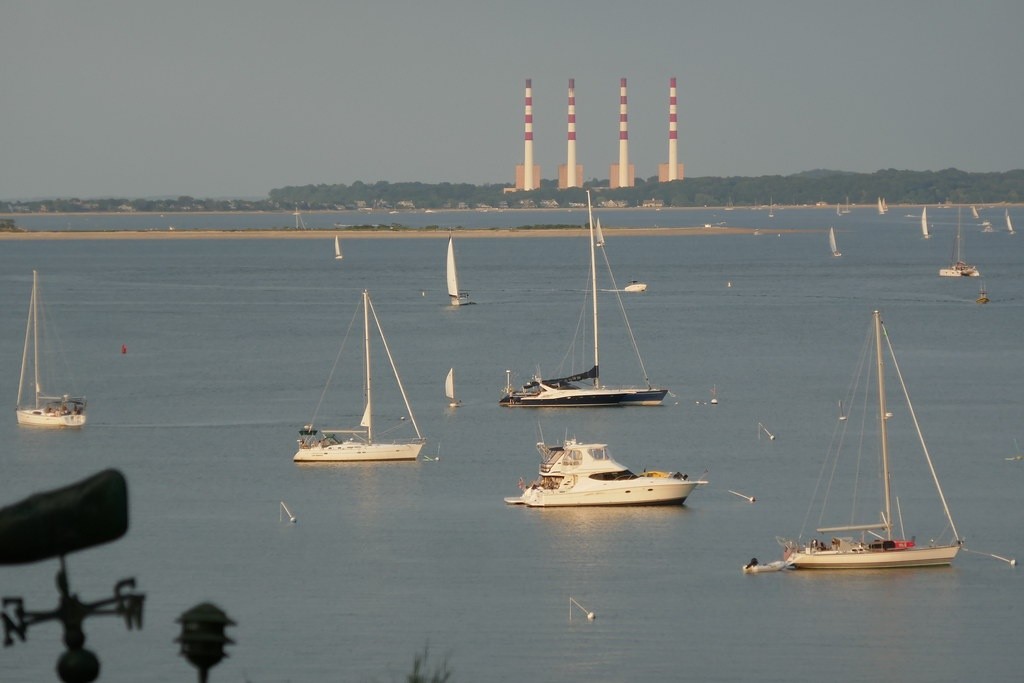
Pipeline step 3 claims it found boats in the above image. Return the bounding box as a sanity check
[504,422,708,508]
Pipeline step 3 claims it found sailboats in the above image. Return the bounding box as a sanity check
[293,289,426,462]
[921,206,934,238]
[445,229,473,305]
[785,309,963,570]
[499,190,668,408]
[334,234,343,260]
[445,368,461,407]
[877,197,888,215]
[828,227,842,257]
[939,210,979,277]
[14,270,86,429]
